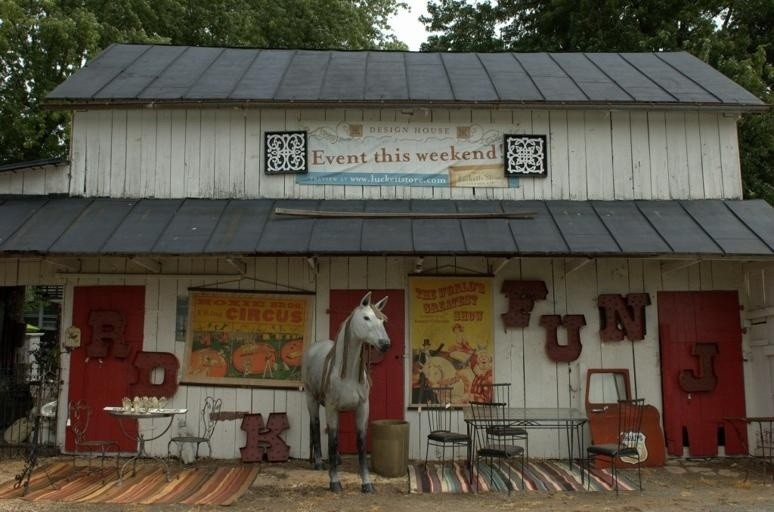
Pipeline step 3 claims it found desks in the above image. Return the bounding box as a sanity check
[729,414,774,483]
[104,406,188,484]
[464,407,588,484]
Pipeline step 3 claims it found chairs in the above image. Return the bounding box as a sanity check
[167,396,222,477]
[421,382,530,494]
[588,398,645,498]
[67,397,120,476]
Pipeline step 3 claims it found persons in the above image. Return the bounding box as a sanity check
[418,323,493,404]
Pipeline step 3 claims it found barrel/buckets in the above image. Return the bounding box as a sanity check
[371,418,410,478]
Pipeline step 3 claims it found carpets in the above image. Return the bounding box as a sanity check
[0,460,259,506]
[404,459,641,494]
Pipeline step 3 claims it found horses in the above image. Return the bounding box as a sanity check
[300,291,391,494]
